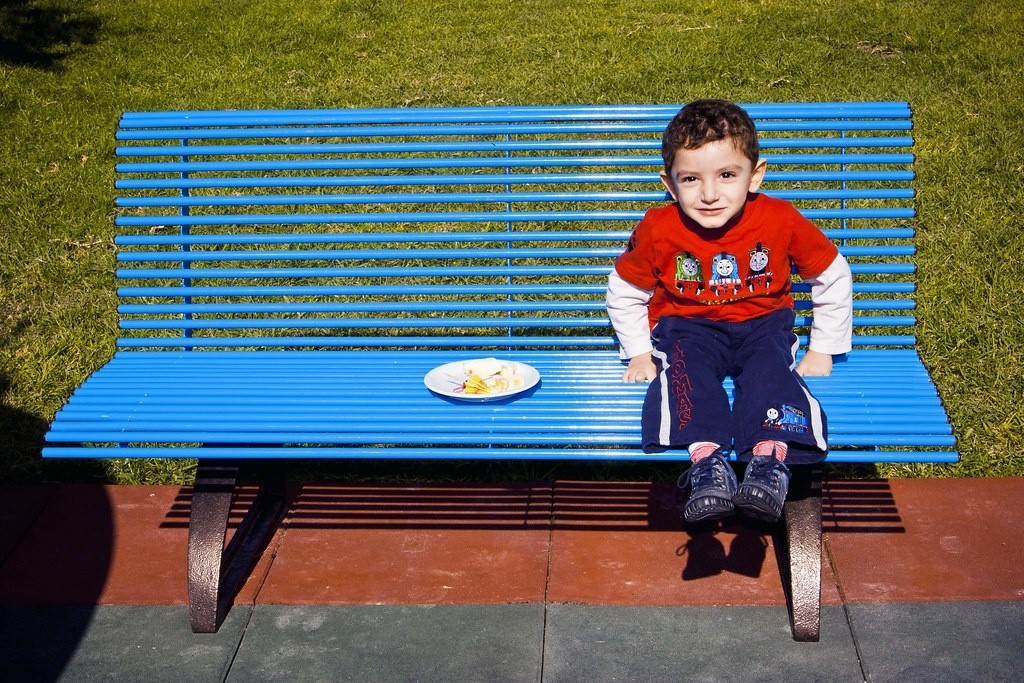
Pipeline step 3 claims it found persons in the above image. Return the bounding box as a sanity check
[606,100,853,523]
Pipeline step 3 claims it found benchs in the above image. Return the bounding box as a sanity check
[42,103,960,644]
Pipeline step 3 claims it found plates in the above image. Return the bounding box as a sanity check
[423,359,540,402]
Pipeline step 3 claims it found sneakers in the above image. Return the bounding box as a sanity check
[731,443,792,523]
[677,445,738,522]
